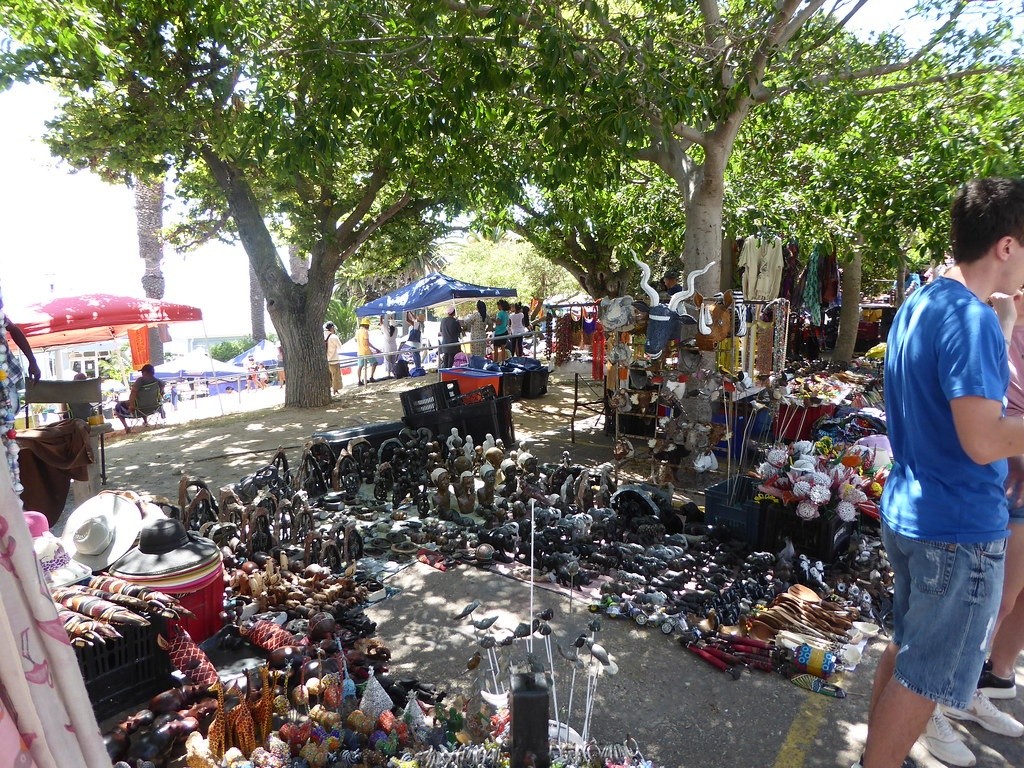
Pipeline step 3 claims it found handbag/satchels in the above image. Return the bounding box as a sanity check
[408,323,420,343]
[410,367,426,377]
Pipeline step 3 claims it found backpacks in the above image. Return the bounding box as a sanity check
[395,360,410,379]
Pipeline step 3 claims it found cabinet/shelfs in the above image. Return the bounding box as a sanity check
[616,298,733,452]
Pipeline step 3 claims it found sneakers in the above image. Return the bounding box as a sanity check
[918,707,977,767]
[942,689,1024,737]
[977,659,1017,698]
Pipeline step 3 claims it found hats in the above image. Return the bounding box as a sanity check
[106,517,225,594]
[31,531,93,589]
[23,511,49,538]
[138,365,155,375]
[62,492,141,571]
[447,308,455,315]
[360,318,370,325]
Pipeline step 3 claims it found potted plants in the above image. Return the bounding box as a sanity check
[87,408,103,425]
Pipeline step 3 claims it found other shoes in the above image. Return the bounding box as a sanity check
[851,751,917,768]
[143,422,147,427]
[358,381,364,385]
[126,427,131,434]
[370,378,378,382]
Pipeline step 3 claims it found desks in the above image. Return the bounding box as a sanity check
[14,422,114,510]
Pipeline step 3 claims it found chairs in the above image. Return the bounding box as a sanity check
[121,381,164,432]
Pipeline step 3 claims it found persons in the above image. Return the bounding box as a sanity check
[245,354,269,391]
[437,307,466,369]
[171,385,178,410]
[324,321,343,396]
[0,287,41,443]
[397,310,426,369]
[226,386,237,394]
[379,314,398,378]
[354,318,377,386]
[275,341,285,388]
[850,173,1024,768]
[430,428,616,517]
[490,299,533,366]
[61,373,96,422]
[663,270,683,296]
[466,300,494,370]
[114,364,161,434]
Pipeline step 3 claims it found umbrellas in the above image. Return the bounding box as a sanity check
[6,285,202,484]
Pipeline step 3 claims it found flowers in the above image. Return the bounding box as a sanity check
[754,436,889,522]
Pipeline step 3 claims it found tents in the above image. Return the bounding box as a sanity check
[542,291,598,331]
[357,271,518,377]
[129,348,249,410]
[225,338,285,367]
